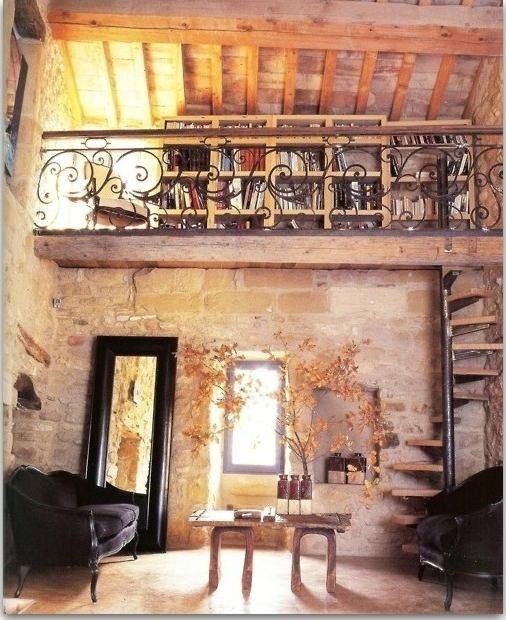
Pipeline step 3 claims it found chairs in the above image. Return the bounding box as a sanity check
[412,463,504,612]
[82,160,151,229]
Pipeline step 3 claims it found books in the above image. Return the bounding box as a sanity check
[217,178,266,210]
[166,122,212,129]
[275,150,324,209]
[162,146,212,171]
[224,122,253,129]
[177,219,202,230]
[221,147,267,171]
[288,219,299,229]
[332,147,382,210]
[162,181,204,210]
[391,133,471,216]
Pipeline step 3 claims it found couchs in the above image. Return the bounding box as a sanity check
[4,466,140,605]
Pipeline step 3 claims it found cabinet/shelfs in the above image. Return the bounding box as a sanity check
[154,112,478,233]
